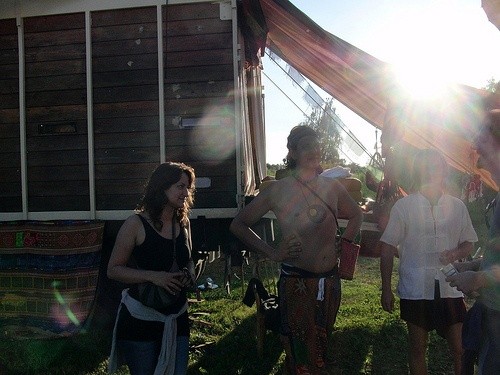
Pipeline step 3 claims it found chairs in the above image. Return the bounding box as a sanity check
[252,257,283,360]
[225,239,265,298]
